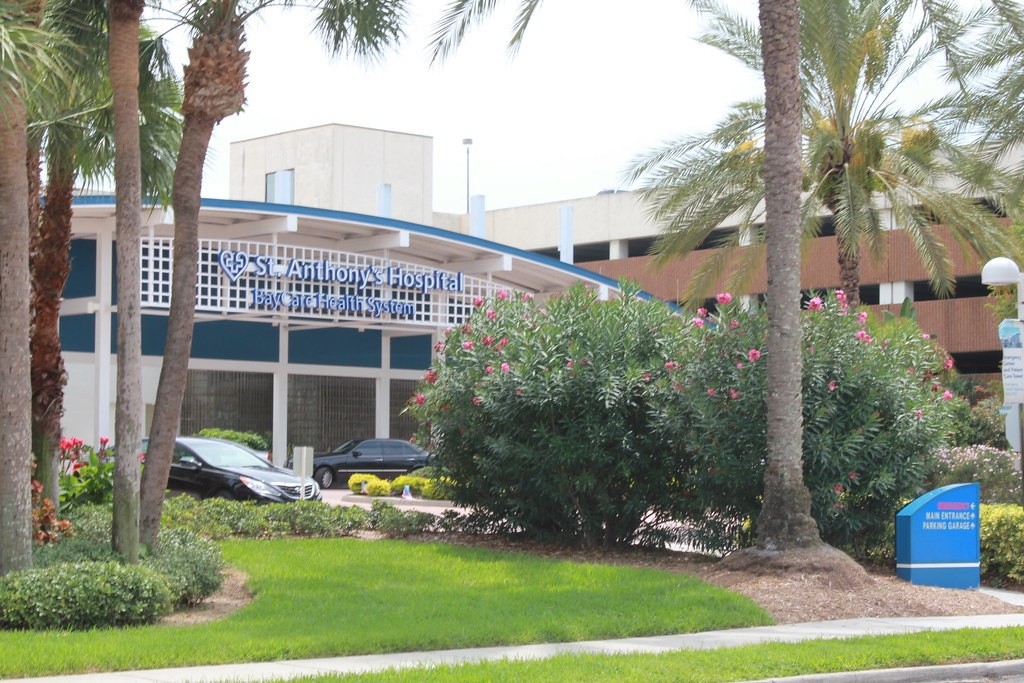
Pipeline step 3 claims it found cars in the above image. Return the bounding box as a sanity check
[95,435,322,507]
[284,439,438,490]
